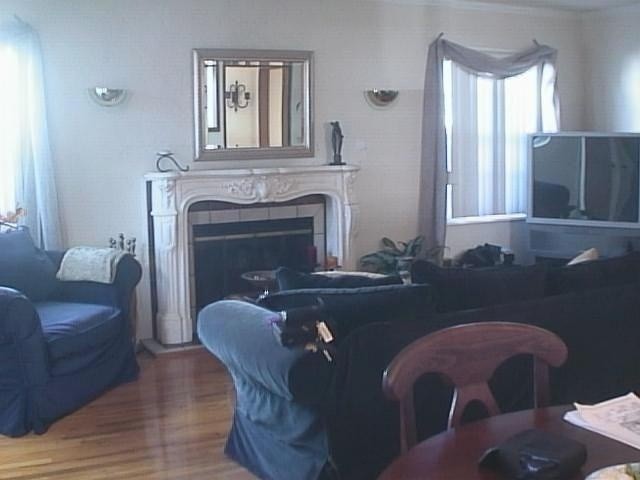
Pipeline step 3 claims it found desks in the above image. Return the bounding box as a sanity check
[374,401,640,480]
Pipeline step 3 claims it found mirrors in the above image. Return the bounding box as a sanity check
[189,43,318,162]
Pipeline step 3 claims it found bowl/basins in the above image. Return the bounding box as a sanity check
[240,270,277,290]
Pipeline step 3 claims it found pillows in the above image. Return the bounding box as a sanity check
[564,245,604,264]
[253,277,436,328]
[269,263,402,294]
[566,250,639,290]
[404,256,604,299]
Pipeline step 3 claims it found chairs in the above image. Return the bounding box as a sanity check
[374,318,572,456]
[0,224,145,445]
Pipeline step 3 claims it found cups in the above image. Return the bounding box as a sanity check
[327,256,337,267]
[307,245,317,265]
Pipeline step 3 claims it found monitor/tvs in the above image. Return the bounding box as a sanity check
[526,131,640,229]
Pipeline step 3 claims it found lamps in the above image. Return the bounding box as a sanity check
[85,86,128,109]
[360,87,401,110]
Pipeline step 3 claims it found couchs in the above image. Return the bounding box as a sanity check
[191,250,640,480]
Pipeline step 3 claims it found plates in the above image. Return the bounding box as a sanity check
[583,460,639,480]
